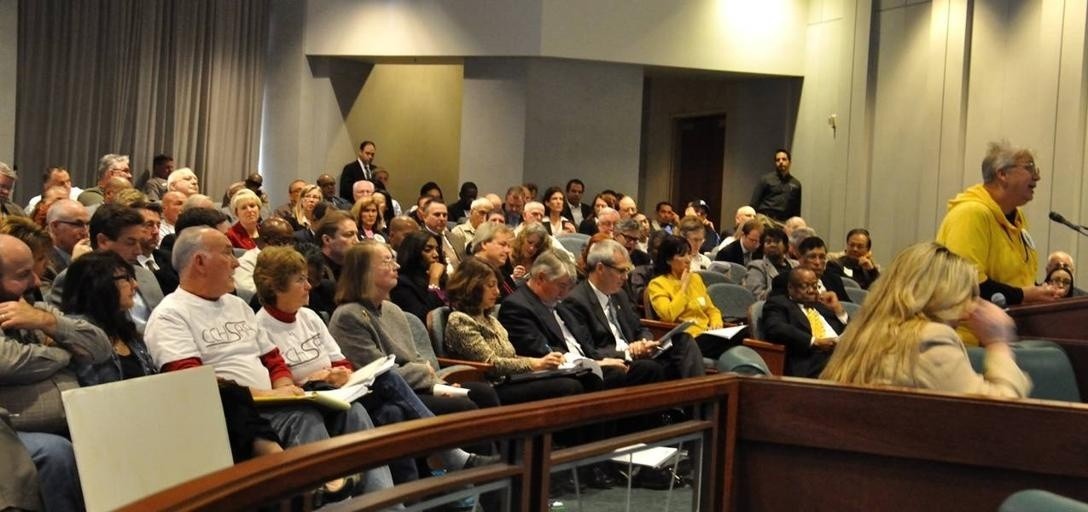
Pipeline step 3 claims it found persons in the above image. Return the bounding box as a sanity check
[815,239,1036,404]
[253,244,500,507]
[928,136,1082,403]
[445,234,853,498]
[1038,266,1075,299]
[328,239,499,457]
[1035,249,1087,298]
[3,139,882,337]
[143,225,406,509]
[3,234,114,510]
[62,250,284,464]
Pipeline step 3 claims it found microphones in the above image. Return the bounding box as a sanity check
[1049,211,1088,237]
[992,292,1010,316]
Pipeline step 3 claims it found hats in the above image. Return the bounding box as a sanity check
[692,199,710,213]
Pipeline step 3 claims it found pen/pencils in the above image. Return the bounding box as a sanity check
[545,344,565,366]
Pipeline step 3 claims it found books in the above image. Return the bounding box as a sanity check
[253,354,397,408]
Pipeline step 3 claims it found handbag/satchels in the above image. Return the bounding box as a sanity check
[608,462,687,490]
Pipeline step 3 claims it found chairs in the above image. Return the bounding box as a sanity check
[425,305,495,376]
[547,228,594,269]
[625,259,868,376]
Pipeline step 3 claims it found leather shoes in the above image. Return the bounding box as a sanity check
[591,476,615,489]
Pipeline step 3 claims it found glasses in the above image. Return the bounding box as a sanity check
[112,271,133,281]
[1051,278,1070,285]
[620,233,640,244]
[748,238,757,244]
[605,264,630,272]
[56,219,85,228]
[1015,163,1039,175]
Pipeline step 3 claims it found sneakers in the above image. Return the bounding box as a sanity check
[463,453,502,469]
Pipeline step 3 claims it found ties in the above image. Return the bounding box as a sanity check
[608,299,630,345]
[804,303,825,338]
[364,166,371,180]
[744,253,750,266]
[439,233,459,269]
